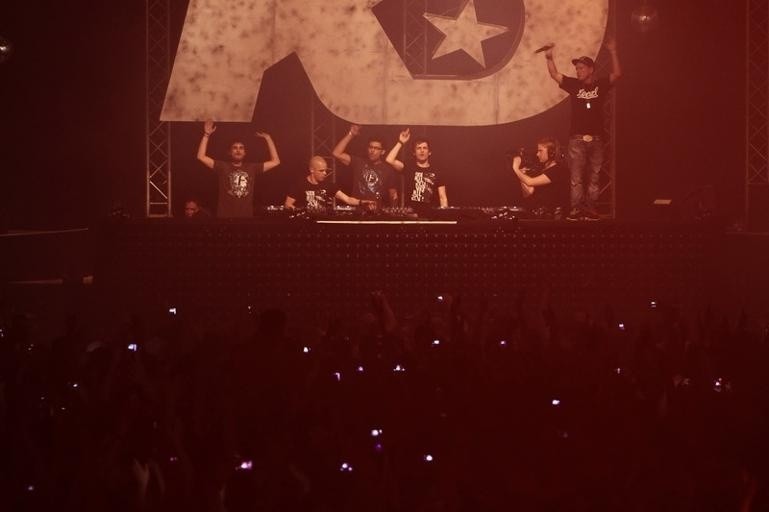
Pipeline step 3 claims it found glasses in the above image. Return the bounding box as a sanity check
[368,146,383,149]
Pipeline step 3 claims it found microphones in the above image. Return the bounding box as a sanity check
[534,42,556,54]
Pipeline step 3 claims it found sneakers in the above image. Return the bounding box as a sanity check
[584,210,601,220]
[567,208,584,221]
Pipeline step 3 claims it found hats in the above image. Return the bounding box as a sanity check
[572,57,595,72]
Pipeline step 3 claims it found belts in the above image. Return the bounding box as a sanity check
[571,135,602,142]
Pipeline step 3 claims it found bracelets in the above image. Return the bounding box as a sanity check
[349,130,354,138]
[359,199,362,206]
[204,131,210,138]
[545,53,553,60]
[397,140,403,146]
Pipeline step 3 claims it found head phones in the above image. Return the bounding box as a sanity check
[548,136,556,162]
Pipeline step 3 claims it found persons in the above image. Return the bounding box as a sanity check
[385,128,448,209]
[182,199,199,217]
[283,156,376,211]
[196,119,280,217]
[332,124,396,209]
[1,286,769,510]
[544,38,622,221]
[512,140,561,208]
